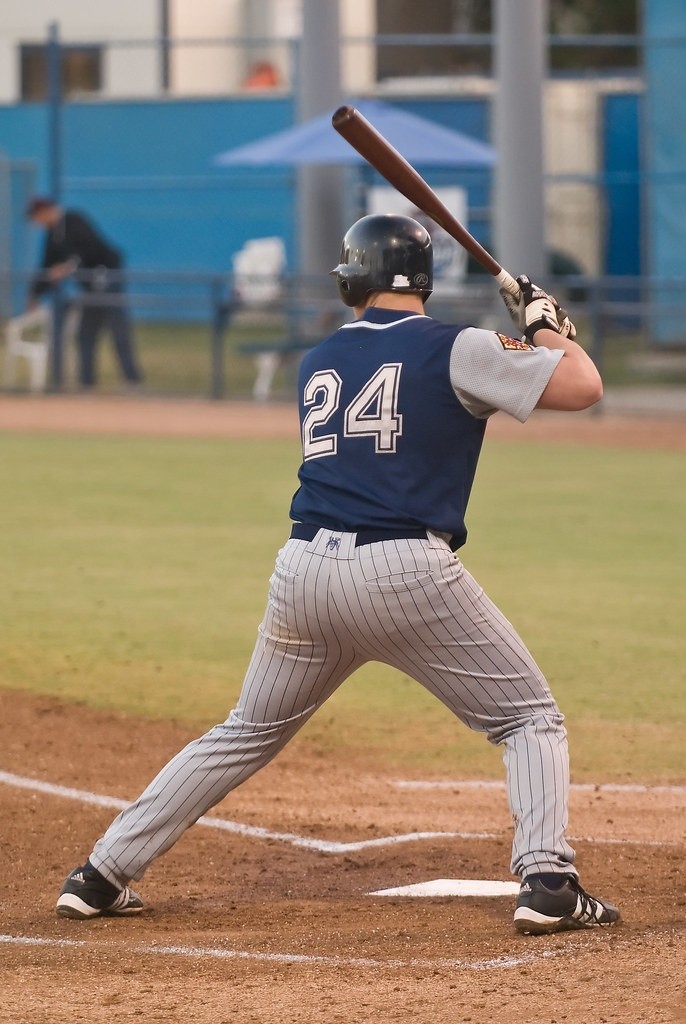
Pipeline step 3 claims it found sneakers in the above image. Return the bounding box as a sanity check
[512,873,620,932]
[55,862,145,918]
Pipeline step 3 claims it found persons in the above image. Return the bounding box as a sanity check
[55,213,625,937]
[24,199,149,390]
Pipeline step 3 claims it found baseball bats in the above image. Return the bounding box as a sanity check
[331,105,524,294]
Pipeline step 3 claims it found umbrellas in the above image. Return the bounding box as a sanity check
[211,95,504,167]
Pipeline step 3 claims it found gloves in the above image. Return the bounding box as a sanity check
[499,276,577,342]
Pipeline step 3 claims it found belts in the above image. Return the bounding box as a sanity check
[289,520,427,550]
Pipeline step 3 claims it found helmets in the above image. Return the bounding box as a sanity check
[329,213,434,306]
